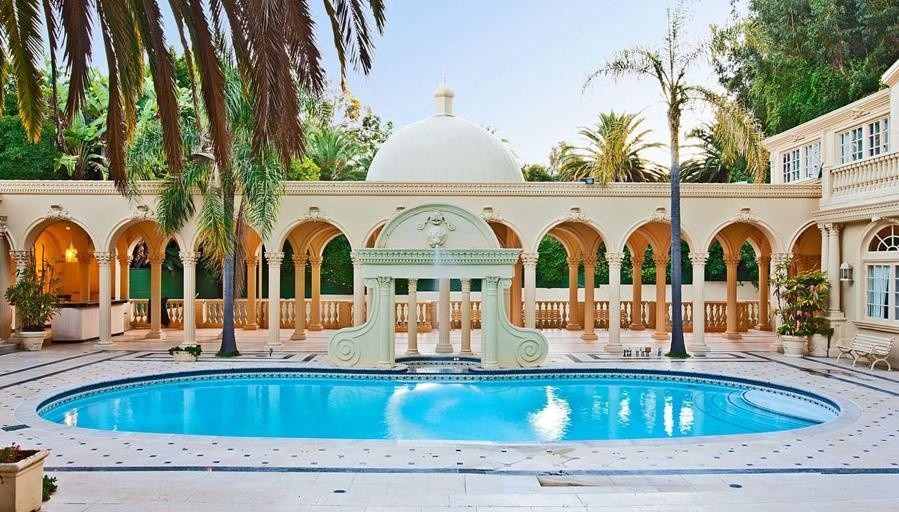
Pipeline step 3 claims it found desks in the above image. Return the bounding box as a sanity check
[50,299,128,343]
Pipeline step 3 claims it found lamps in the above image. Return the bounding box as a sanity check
[838,261,853,283]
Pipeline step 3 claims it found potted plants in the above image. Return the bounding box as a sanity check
[767,251,835,358]
[2,248,66,353]
[0,441,50,511]
[168,345,203,363]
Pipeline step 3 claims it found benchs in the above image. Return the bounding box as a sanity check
[835,331,896,374]
